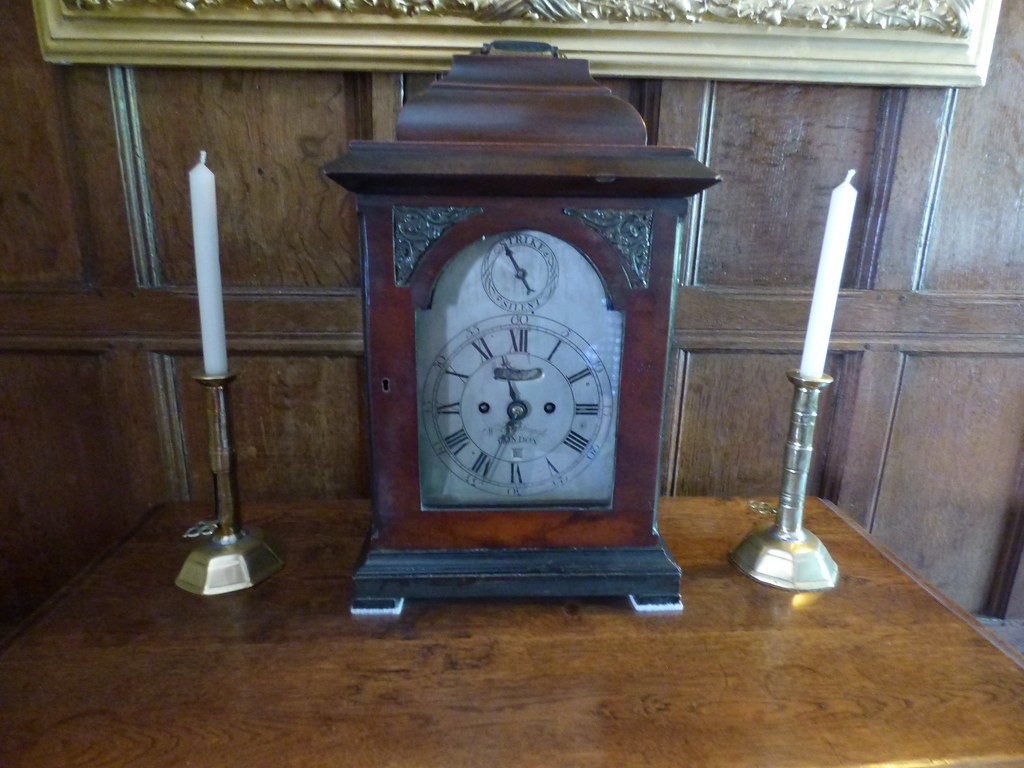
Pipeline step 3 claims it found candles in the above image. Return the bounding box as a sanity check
[188,151,229,377]
[798,169,857,379]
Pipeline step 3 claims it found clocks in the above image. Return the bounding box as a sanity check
[424,231,613,500]
